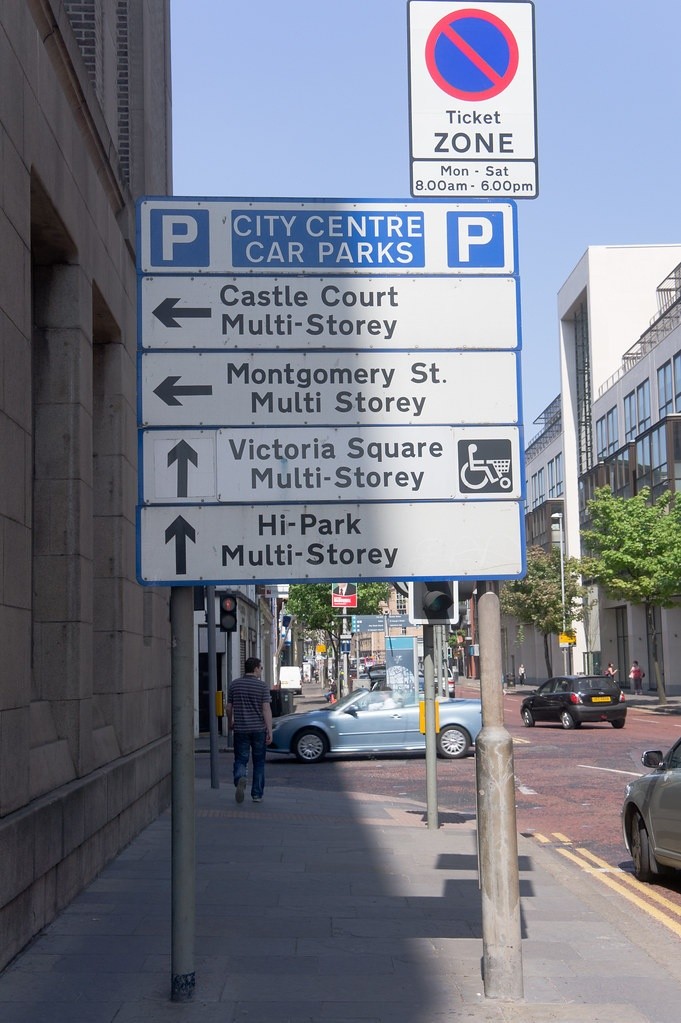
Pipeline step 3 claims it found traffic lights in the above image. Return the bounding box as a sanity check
[219,594,238,632]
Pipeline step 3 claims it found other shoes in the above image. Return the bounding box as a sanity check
[639,690,643,694]
[235,778,247,804]
[253,796,262,802]
[635,690,638,695]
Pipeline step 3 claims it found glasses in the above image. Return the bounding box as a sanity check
[259,667,264,670]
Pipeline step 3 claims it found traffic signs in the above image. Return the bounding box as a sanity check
[133,195,528,587]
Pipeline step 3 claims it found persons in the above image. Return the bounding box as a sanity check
[324,679,337,703]
[605,661,618,679]
[502,673,507,695]
[226,657,273,803]
[630,660,643,695]
[519,663,525,686]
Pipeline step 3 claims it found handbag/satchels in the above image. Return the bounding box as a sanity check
[524,672,526,679]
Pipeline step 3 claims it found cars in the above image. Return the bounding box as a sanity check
[330,657,425,692]
[434,668,455,698]
[520,675,628,730]
[267,689,483,763]
[619,737,681,882]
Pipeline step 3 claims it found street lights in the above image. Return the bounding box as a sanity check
[552,513,567,675]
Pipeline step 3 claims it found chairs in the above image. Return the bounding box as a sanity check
[395,702,404,709]
